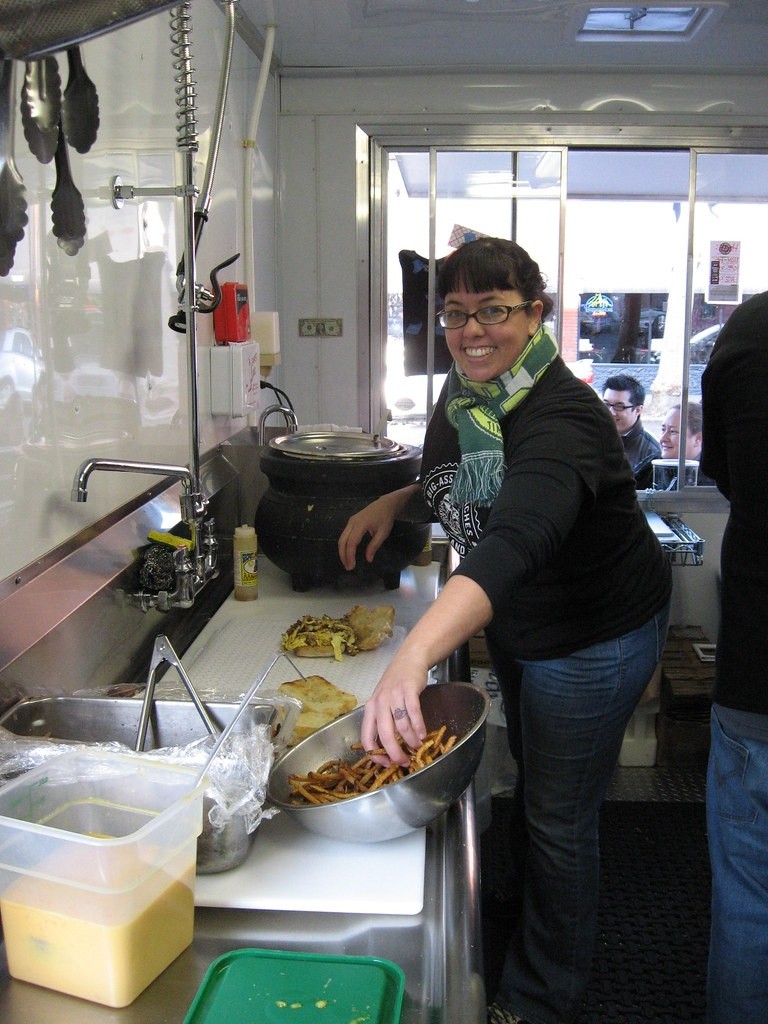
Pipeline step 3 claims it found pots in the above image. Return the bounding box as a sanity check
[255,441,431,592]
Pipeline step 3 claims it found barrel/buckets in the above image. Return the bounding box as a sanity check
[1,750,214,1008]
[651,459,700,492]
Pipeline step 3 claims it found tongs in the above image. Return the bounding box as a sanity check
[50,46,99,257]
[136,634,218,752]
[0,54,62,279]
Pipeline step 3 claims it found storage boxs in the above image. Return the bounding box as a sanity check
[0,752,203,1007]
[0,693,278,881]
[252,432,441,592]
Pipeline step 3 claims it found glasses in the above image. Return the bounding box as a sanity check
[603,403,640,411]
[435,300,534,329]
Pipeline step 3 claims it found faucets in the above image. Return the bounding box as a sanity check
[256,404,301,449]
[70,457,205,518]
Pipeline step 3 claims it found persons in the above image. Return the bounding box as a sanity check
[338,231,682,1020]
[626,400,716,489]
[602,372,666,492]
[696,284,766,1024]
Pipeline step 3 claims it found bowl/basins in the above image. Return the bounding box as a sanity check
[267,681,492,843]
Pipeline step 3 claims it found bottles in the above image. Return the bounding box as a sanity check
[233,524,258,601]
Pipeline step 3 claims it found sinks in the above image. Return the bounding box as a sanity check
[3,691,290,792]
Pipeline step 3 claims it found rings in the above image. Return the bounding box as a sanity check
[392,706,407,722]
